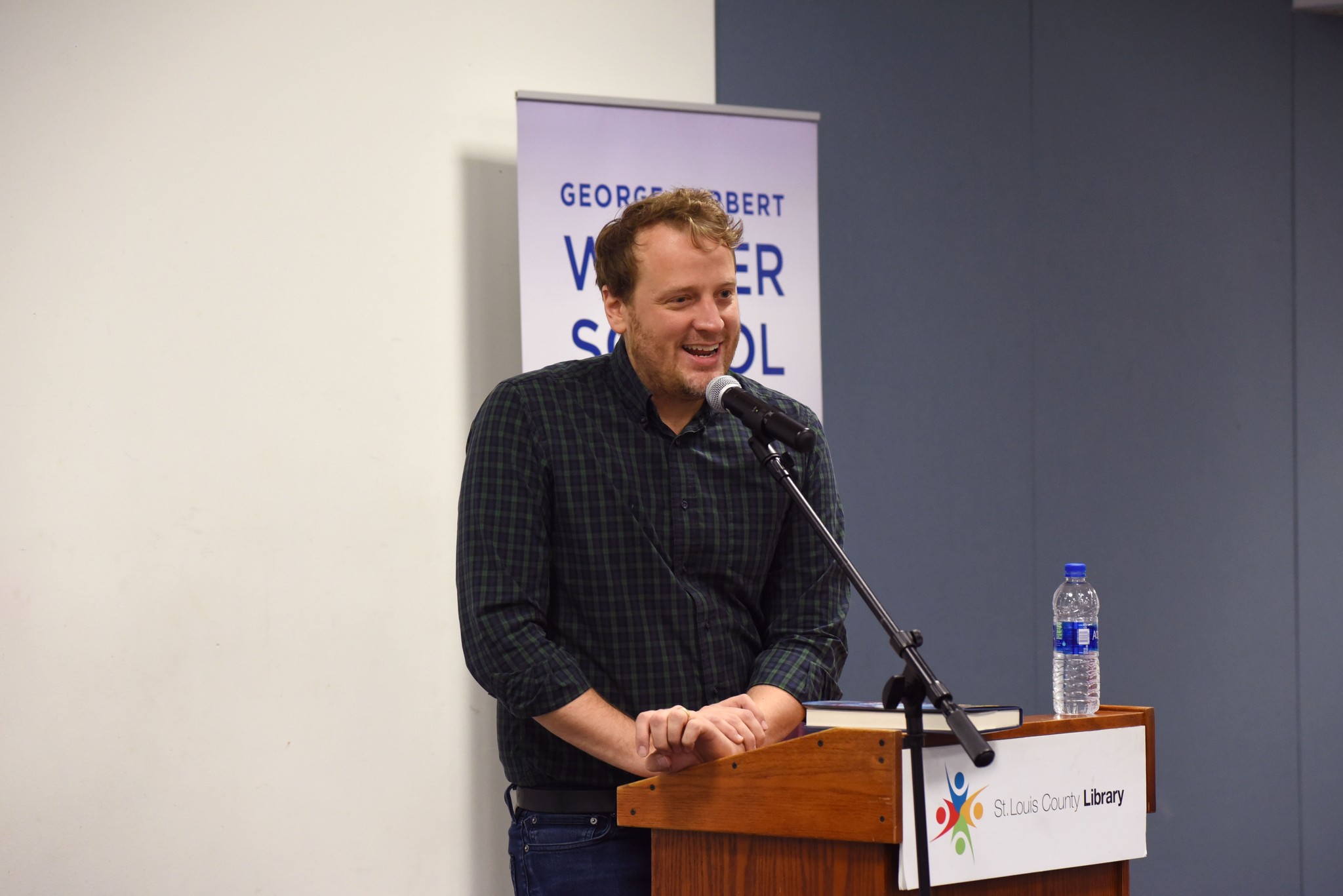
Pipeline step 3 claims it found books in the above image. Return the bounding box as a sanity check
[802,701,1023,737]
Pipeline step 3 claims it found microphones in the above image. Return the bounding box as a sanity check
[706,375,816,453]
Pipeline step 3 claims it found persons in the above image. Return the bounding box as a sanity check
[457,188,848,896]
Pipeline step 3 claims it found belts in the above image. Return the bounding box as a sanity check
[515,785,617,814]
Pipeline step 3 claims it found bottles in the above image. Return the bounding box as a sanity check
[1053,562,1101,716]
